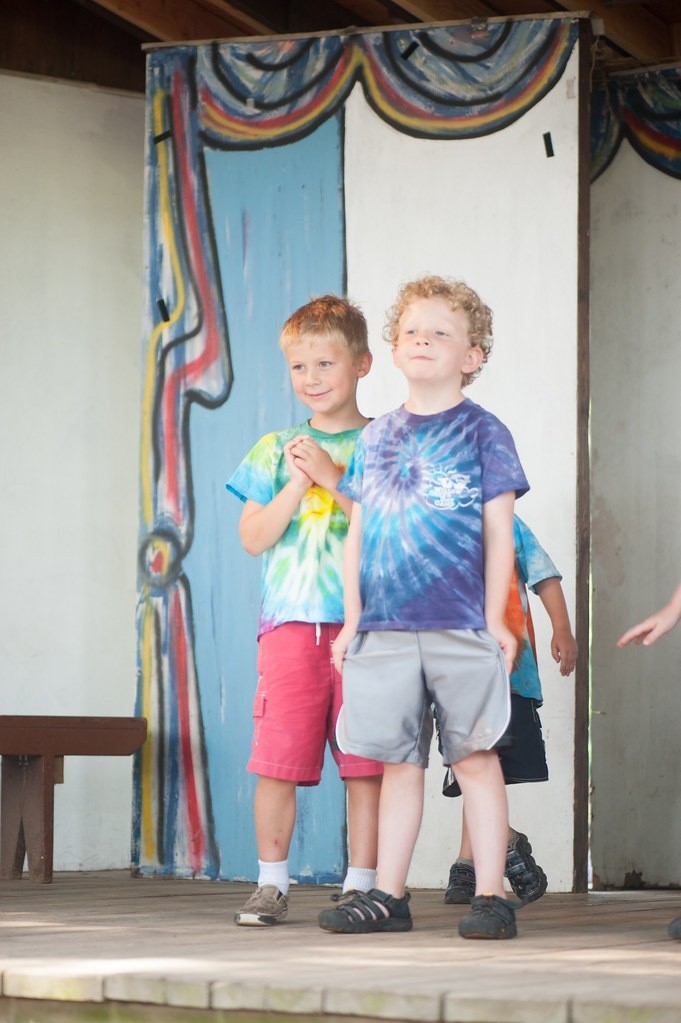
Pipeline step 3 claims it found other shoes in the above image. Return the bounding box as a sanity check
[667,916,681,940]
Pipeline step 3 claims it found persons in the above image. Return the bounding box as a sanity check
[224,273,575,940]
[614,582,681,649]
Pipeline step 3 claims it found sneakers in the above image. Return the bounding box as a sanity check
[337,890,365,907]
[505,831,549,902]
[444,862,476,904]
[234,885,289,926]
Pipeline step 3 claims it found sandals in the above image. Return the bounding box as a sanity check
[458,894,525,939]
[318,888,412,933]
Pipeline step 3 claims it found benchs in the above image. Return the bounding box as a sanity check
[0,714,147,884]
